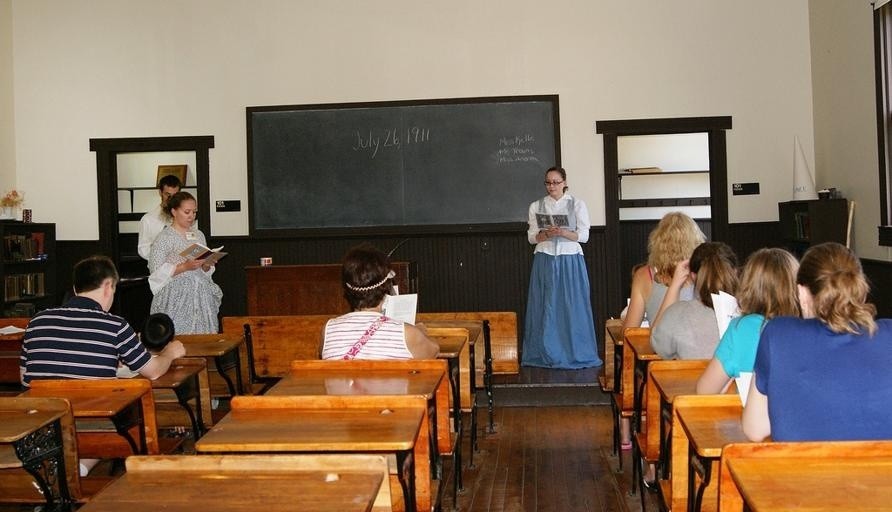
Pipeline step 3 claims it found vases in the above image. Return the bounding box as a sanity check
[0,209,18,219]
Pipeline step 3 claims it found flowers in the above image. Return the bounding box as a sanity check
[2,188,26,208]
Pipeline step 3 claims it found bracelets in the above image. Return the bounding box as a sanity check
[546,231,551,239]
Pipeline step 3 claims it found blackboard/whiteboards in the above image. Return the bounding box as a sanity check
[246,95,561,237]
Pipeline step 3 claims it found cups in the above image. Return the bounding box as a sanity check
[23,208,32,223]
[259,256,273,267]
[818,188,831,198]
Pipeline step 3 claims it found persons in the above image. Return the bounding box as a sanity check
[519,166,604,369]
[621,212,707,338]
[318,248,439,363]
[621,262,648,321]
[741,243,891,443]
[147,191,230,410]
[695,247,800,395]
[19,255,186,477]
[138,175,181,260]
[116,312,175,378]
[649,241,740,360]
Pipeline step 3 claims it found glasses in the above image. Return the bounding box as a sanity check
[544,180,564,186]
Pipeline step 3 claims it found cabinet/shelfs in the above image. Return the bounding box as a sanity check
[1,220,58,315]
[778,198,849,264]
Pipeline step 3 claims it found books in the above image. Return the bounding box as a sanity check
[630,167,664,173]
[710,291,738,340]
[2,233,49,303]
[179,244,228,266]
[384,294,419,324]
[531,213,570,229]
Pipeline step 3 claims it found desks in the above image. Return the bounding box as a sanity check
[80,311,495,509]
[599,310,892,511]
[2,310,247,512]
